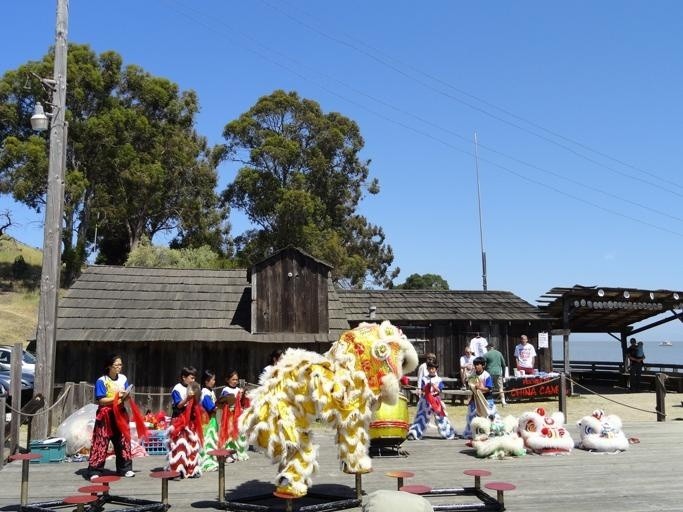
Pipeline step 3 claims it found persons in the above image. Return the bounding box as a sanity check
[514,335,537,375]
[417,353,437,399]
[627,342,647,371]
[218,371,249,462]
[198,369,220,472]
[459,343,476,391]
[482,343,508,407]
[407,357,459,440]
[461,356,499,439]
[164,366,205,481]
[87,355,136,482]
[627,338,641,393]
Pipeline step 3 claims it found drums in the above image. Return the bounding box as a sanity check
[368,394,408,443]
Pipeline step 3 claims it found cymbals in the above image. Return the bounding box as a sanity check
[429,378,432,391]
[215,393,236,409]
[121,384,136,401]
[187,382,201,406]
[241,388,249,407]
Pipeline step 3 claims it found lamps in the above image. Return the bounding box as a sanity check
[594,288,679,302]
[29,99,60,131]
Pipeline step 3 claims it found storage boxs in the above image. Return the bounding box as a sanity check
[30,441,66,463]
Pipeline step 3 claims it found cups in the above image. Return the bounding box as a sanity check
[512,367,559,377]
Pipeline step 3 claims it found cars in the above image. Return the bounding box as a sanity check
[0,343,36,374]
[0,368,35,397]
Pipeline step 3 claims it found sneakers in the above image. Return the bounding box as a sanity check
[225,456,234,463]
[125,471,135,478]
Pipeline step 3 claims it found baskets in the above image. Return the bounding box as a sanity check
[141,435,168,455]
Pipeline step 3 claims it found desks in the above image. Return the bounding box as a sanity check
[505,371,570,402]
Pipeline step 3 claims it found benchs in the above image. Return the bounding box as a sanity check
[621,371,683,393]
[402,374,473,407]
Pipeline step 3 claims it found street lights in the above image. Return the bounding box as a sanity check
[27,100,67,444]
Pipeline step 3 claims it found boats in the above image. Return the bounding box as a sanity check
[657,339,673,347]
